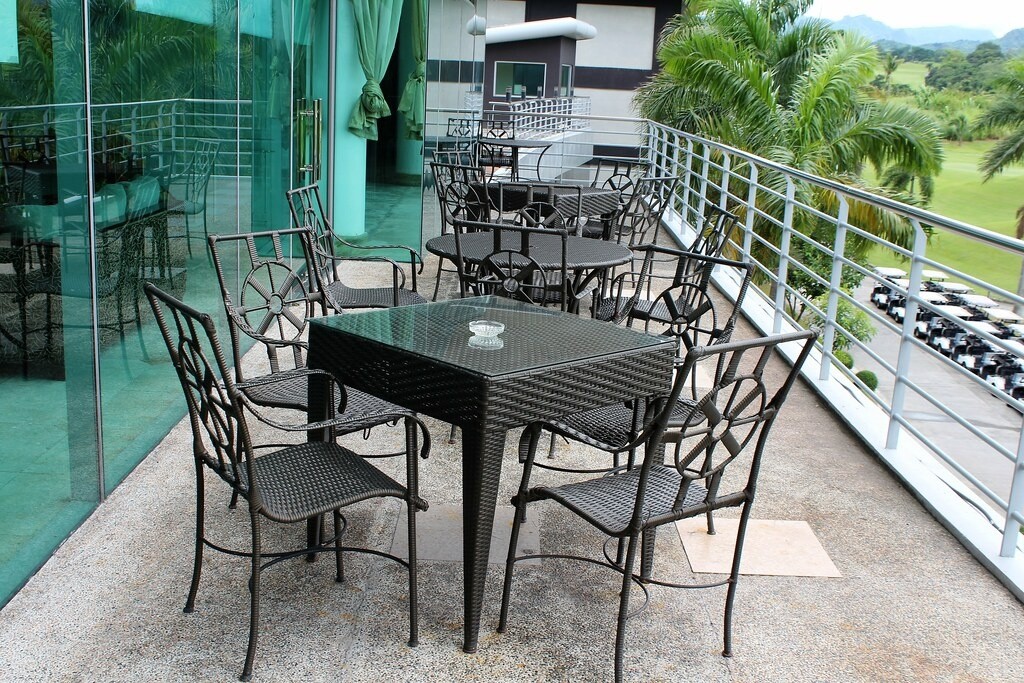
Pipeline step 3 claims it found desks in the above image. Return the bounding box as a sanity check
[479,138,553,186]
[300,295,680,653]
[424,135,478,193]
[463,177,623,311]
[426,231,635,320]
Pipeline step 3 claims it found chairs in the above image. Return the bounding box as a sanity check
[2,128,220,386]
[447,217,573,449]
[578,177,679,253]
[498,327,820,682]
[442,118,482,179]
[286,185,429,317]
[592,204,741,372]
[431,150,499,224]
[495,182,583,305]
[207,227,418,508]
[139,279,430,681]
[543,241,756,477]
[478,118,520,183]
[568,156,650,237]
[430,160,522,280]
